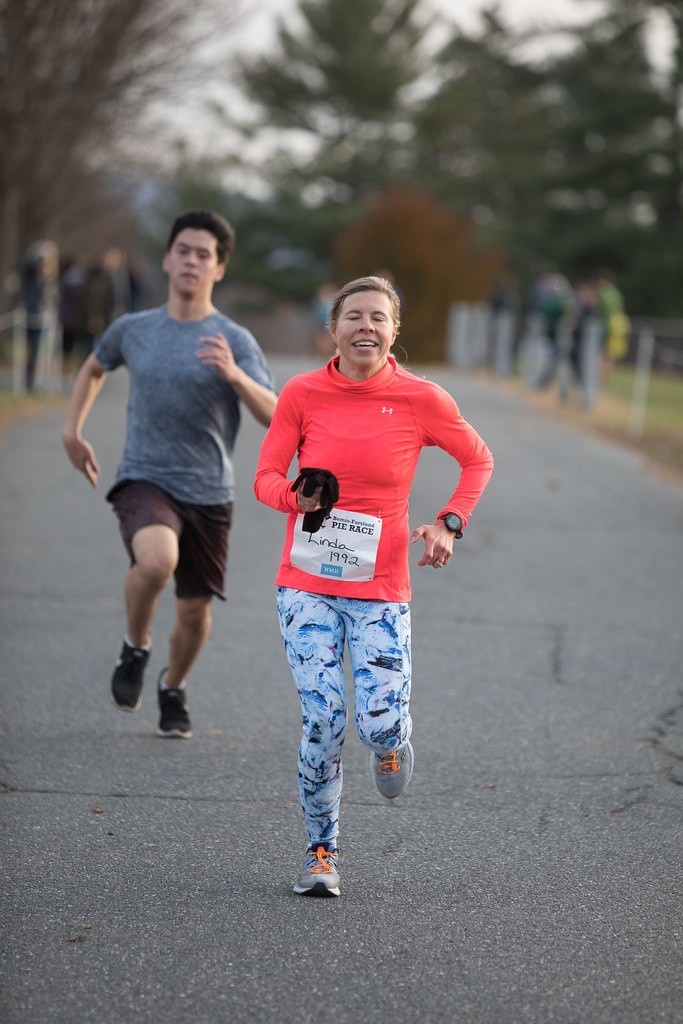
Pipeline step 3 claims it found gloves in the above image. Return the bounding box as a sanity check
[290,468,339,533]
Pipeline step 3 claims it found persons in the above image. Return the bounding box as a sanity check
[23,238,159,400]
[490,266,633,400]
[253,275,494,895]
[61,210,282,737]
[310,280,341,359]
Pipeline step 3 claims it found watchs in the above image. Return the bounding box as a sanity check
[440,514,463,532]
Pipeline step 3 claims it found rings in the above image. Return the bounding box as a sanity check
[435,560,444,567]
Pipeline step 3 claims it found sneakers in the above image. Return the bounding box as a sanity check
[154,666,194,739]
[370,713,415,800]
[293,840,342,897]
[109,634,153,712]
